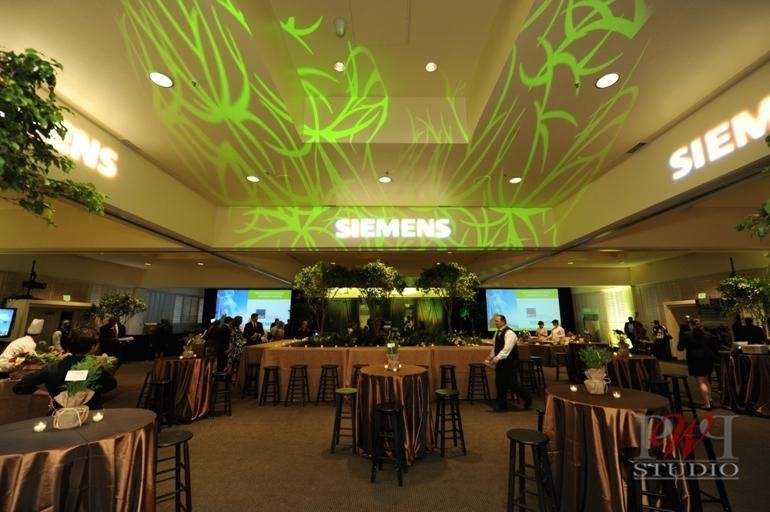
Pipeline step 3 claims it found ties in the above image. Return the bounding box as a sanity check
[254,323,256,329]
[496,330,500,336]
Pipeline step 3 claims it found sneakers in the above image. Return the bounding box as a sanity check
[494,396,533,412]
[700,401,713,410]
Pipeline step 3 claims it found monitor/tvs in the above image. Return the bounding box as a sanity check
[0,307,18,337]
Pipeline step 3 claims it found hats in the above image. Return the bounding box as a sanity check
[27,318,45,336]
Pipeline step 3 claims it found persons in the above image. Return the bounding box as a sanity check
[51,319,70,353]
[99,315,130,377]
[546,319,566,368]
[1,331,41,380]
[346,315,427,347]
[200,313,312,387]
[535,320,550,367]
[12,327,117,417]
[484,314,533,412]
[624,314,766,414]
